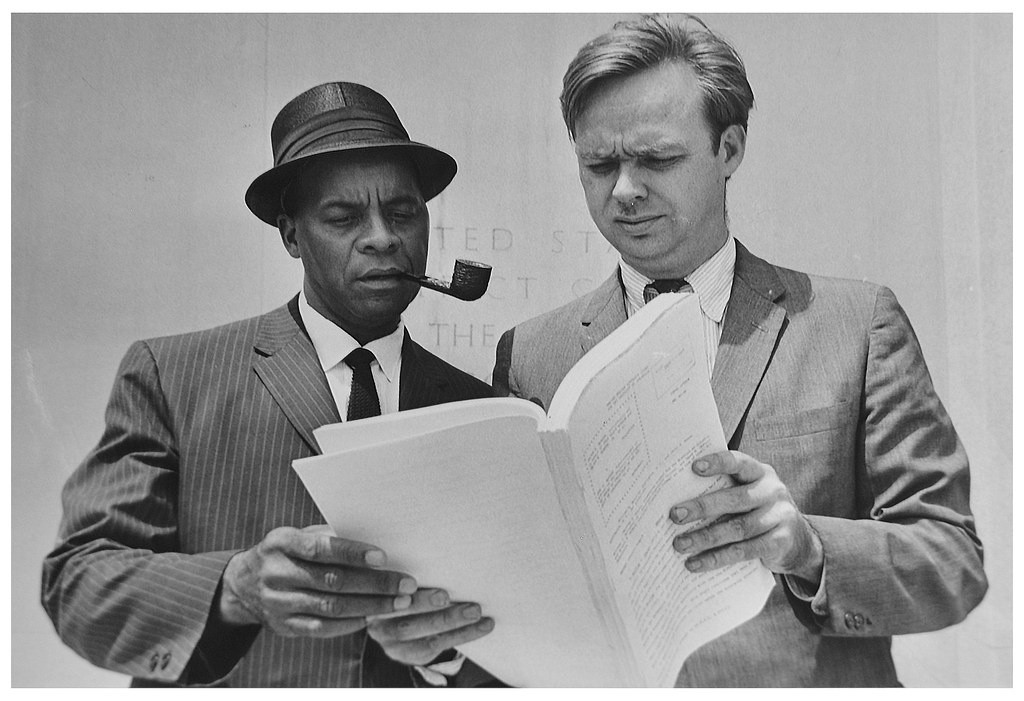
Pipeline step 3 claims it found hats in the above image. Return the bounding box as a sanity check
[243,81,458,229]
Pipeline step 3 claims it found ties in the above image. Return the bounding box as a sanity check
[646,279,688,294]
[339,349,381,422]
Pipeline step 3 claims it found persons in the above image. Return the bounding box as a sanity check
[38,81,495,688]
[366,12,989,688]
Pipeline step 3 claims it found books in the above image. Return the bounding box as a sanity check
[290,292,777,688]
